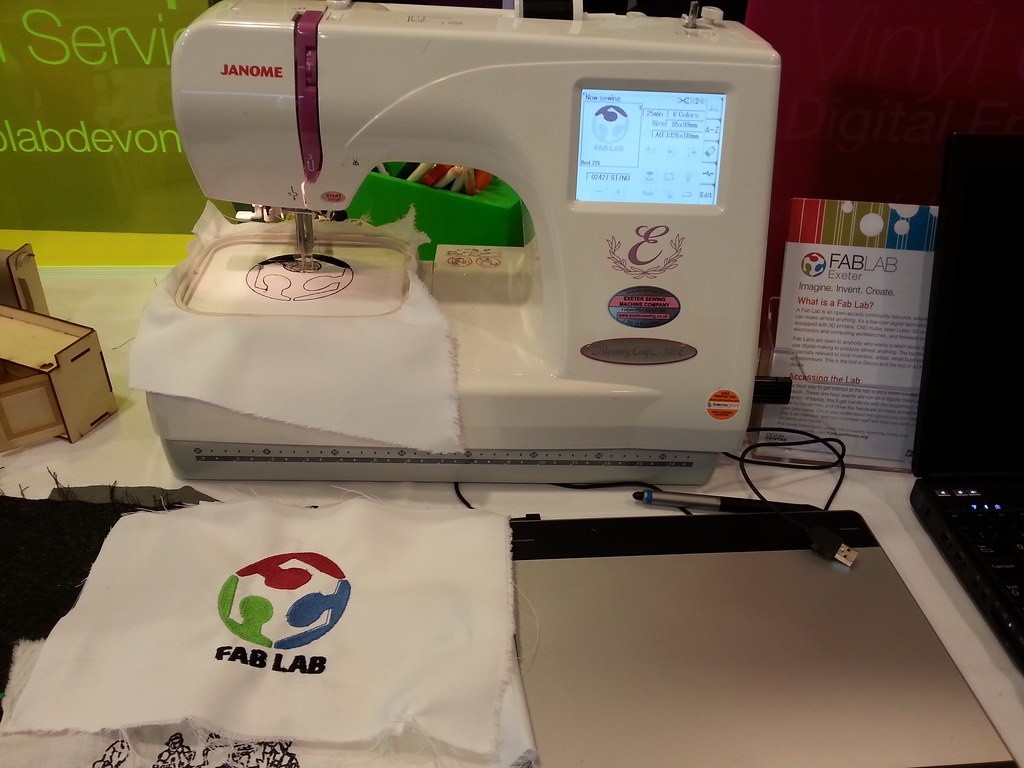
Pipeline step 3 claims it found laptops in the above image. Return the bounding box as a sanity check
[910,130,1024,676]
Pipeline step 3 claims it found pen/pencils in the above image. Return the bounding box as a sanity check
[406,163,493,196]
[630,486,827,513]
[377,164,390,177]
